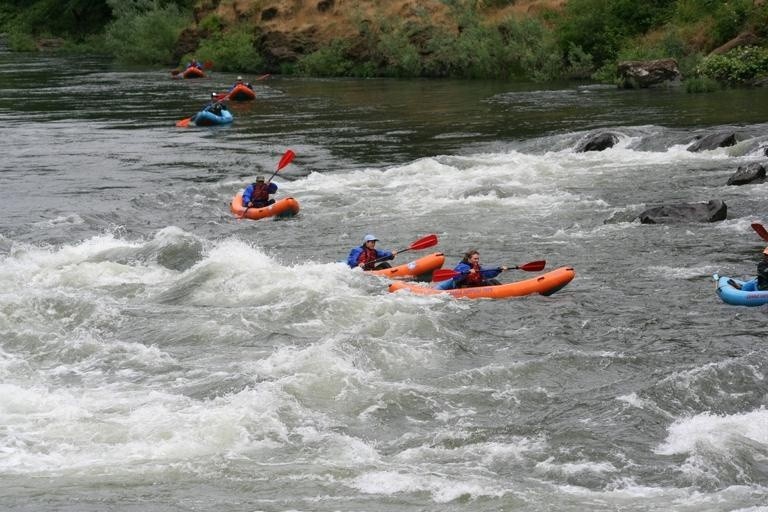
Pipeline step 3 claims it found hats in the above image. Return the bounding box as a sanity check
[256,175,264,182]
[236,76,243,81]
[365,235,379,241]
[211,92,219,97]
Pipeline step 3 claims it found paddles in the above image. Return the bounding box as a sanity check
[250,74,272,85]
[363,235,437,269]
[177,93,235,128]
[238,150,296,219]
[429,260,545,283]
[751,223,768,243]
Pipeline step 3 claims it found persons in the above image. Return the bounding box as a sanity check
[346,233,397,269]
[756,246,768,292]
[439,250,506,290]
[204,92,227,114]
[185,59,201,68]
[241,176,277,208]
[227,76,254,91]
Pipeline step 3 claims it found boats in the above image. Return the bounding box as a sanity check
[712,273,768,306]
[195,109,232,126]
[387,266,575,301]
[362,252,445,281]
[183,67,205,80]
[229,191,300,219]
[226,84,256,101]
